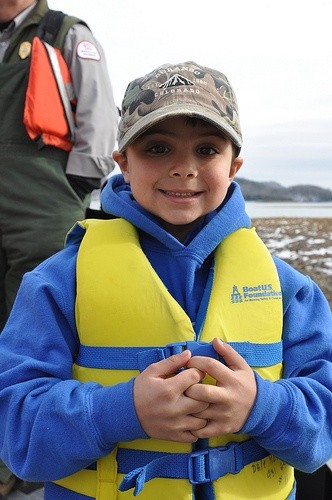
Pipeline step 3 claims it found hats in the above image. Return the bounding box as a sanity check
[117,61,243,158]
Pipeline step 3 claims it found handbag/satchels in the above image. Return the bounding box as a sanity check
[23,36,77,150]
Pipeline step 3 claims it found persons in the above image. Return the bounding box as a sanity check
[0,1,119,500]
[0,63,332,500]
